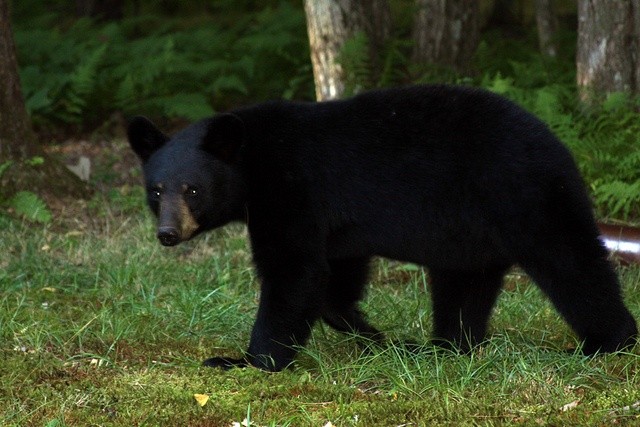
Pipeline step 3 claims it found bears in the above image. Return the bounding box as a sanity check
[121,82,638,374]
